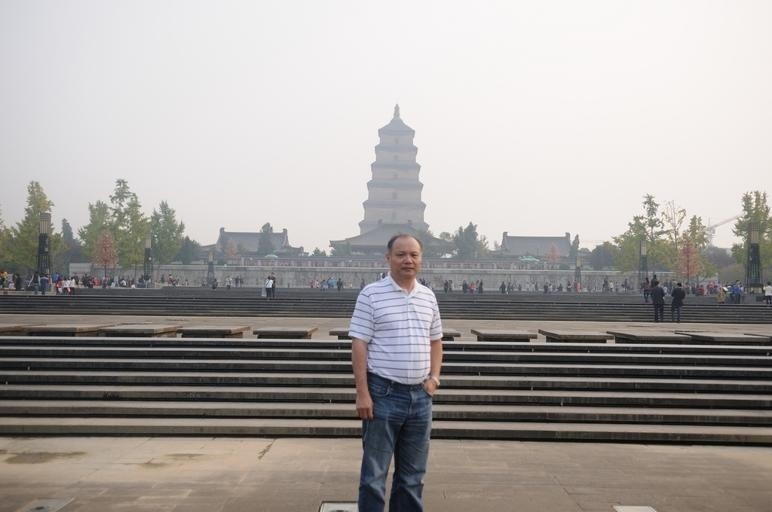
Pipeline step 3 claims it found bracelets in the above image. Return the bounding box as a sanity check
[431,375,441,387]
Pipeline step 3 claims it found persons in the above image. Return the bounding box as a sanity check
[0,270,245,297]
[652,281,665,322]
[264,272,277,301]
[308,277,365,292]
[348,234,444,511]
[671,282,685,323]
